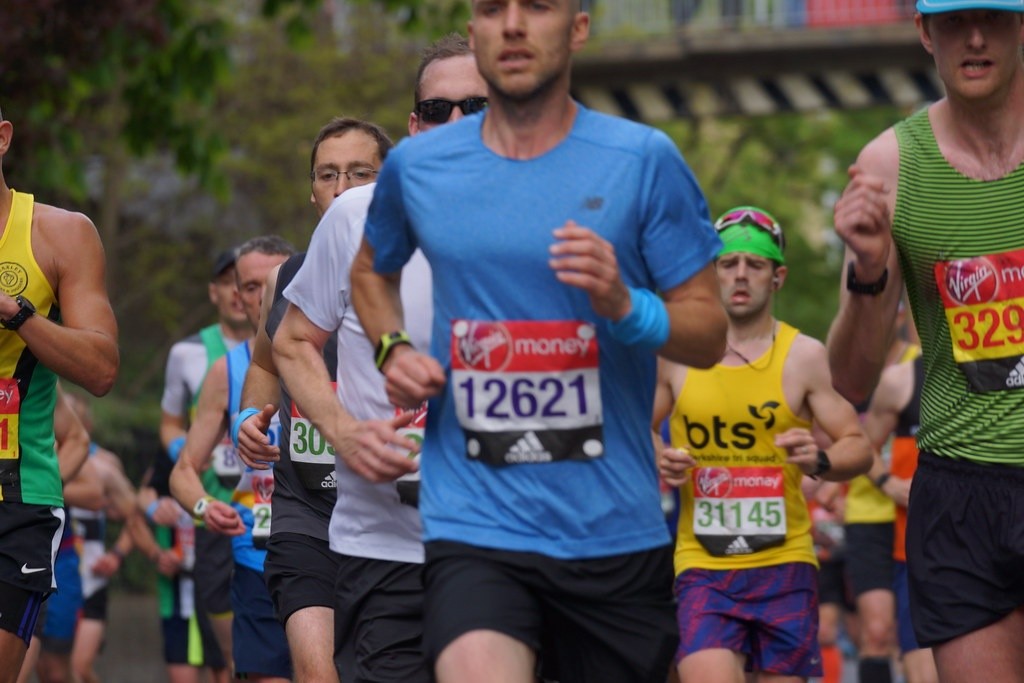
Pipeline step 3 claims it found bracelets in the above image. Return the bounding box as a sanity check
[146,502,158,518]
[232,407,258,448]
[847,261,888,295]
[112,547,123,562]
[878,474,890,488]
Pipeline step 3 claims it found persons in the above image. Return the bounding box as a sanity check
[353,0,728,683]
[15,244,252,683]
[652,205,923,683]
[170,239,295,683]
[272,32,489,683]
[827,0,1024,682]
[0,111,119,683]
[232,117,392,683]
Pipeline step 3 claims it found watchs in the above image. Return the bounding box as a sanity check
[1,296,36,331]
[373,331,415,372]
[810,450,832,481]
[194,496,214,519]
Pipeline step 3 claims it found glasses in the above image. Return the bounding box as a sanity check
[311,167,378,182]
[413,96,490,124]
[715,210,780,236]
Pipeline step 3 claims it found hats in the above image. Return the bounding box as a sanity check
[916,0,1024,14]
[211,247,240,278]
